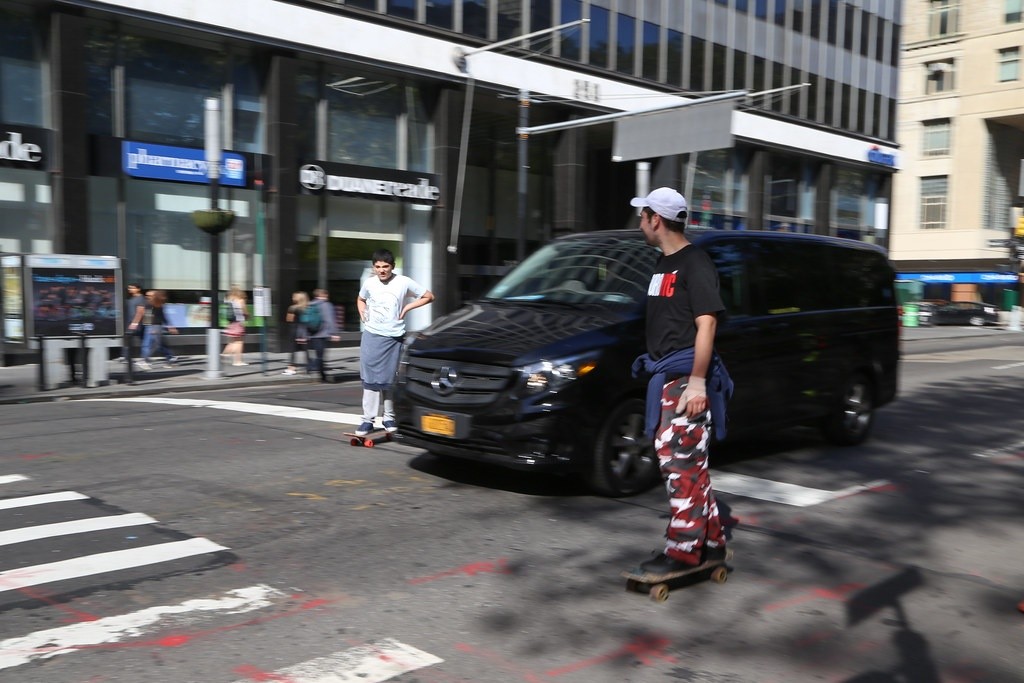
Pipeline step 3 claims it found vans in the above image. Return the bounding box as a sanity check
[389,221,905,501]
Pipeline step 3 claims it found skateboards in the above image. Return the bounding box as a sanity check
[342,427,397,447]
[620,544,734,602]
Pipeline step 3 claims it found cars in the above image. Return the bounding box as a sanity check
[919,301,999,327]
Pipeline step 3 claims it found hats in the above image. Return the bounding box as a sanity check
[629,186,688,223]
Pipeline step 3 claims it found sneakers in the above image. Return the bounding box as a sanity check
[641,553,690,573]
[355,422,374,436]
[384,421,398,432]
[701,545,727,560]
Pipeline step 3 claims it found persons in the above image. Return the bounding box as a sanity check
[628,185,731,574]
[121,284,180,368]
[349,253,436,436]
[216,284,252,366]
[282,288,340,381]
